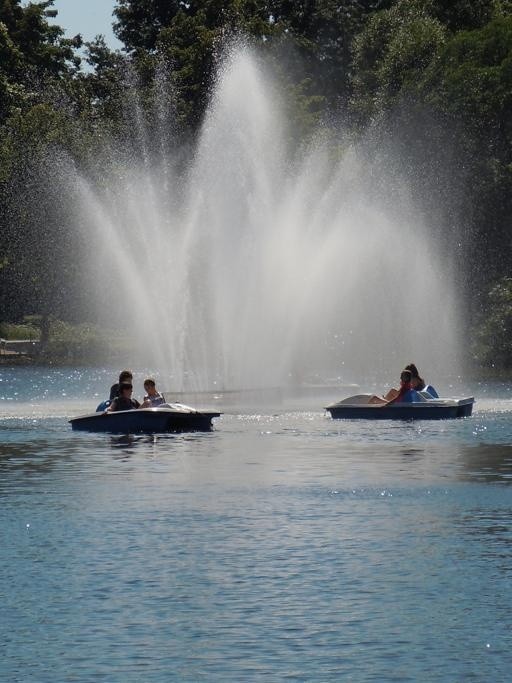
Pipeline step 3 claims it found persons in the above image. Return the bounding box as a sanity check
[101,381,136,414]
[366,370,414,404]
[109,369,141,409]
[383,363,424,401]
[143,378,166,406]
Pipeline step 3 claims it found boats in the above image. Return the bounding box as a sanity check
[323,390,477,421]
[65,398,225,431]
